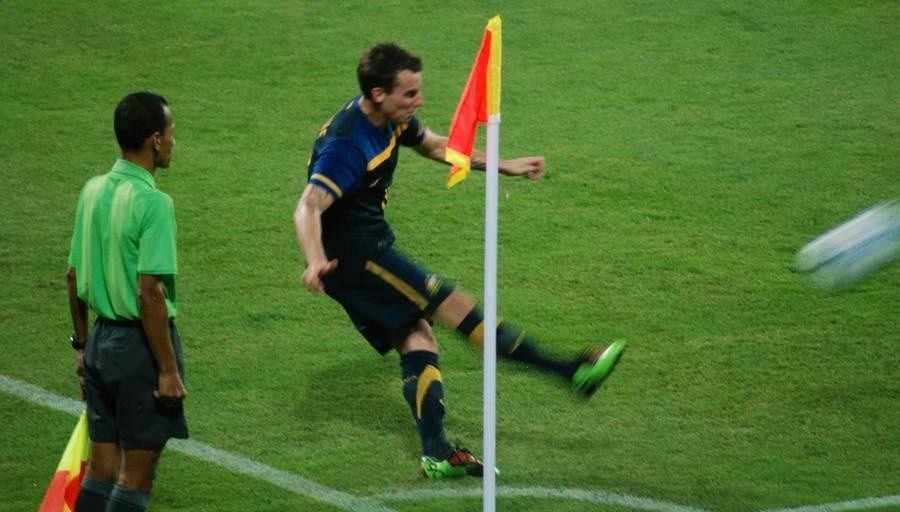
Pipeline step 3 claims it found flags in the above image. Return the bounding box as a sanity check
[439,14,500,188]
[37,407,92,511]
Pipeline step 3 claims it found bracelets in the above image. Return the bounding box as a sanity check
[71,335,88,349]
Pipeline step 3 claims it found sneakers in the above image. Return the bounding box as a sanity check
[420,446,500,481]
[569,339,625,397]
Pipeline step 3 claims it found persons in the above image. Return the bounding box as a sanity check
[68,90,190,511]
[293,42,630,480]
